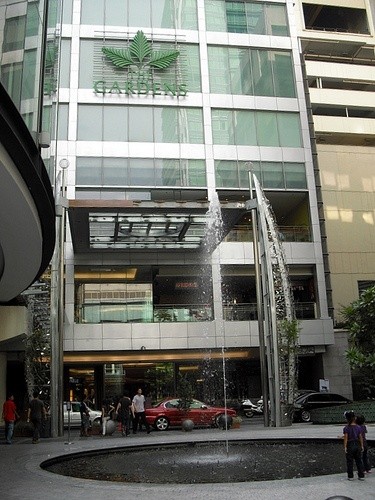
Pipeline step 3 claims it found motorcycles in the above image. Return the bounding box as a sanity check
[241,394,271,418]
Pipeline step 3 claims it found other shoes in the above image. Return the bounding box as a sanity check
[347,477,354,481]
[32,441,37,444]
[38,439,40,443]
[369,469,372,473]
[364,471,367,473]
[358,477,365,480]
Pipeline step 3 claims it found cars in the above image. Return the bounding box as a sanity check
[144,396,238,431]
[281,390,316,405]
[281,392,375,423]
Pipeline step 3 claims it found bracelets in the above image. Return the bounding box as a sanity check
[28,417,29,418]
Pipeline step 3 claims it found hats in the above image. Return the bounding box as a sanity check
[137,389,143,394]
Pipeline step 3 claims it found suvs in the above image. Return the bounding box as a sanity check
[45,401,102,429]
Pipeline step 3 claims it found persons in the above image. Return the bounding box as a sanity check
[337,415,372,473]
[343,411,366,480]
[131,388,151,434]
[2,393,20,444]
[77,394,90,437]
[116,394,132,436]
[26,391,47,444]
